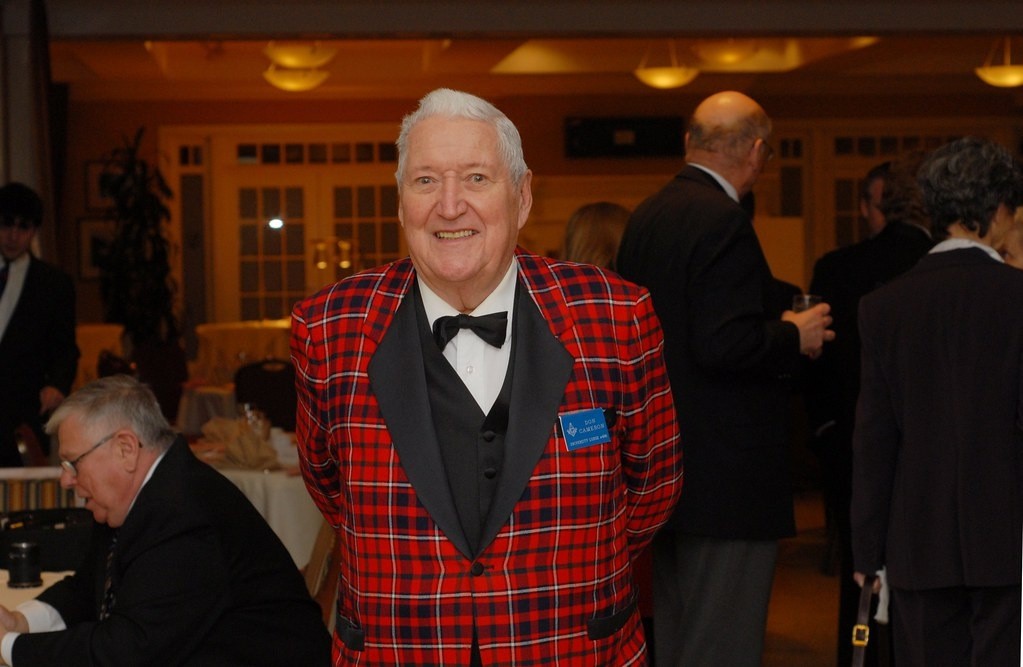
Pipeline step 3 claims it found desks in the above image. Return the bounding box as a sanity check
[191,431,322,583]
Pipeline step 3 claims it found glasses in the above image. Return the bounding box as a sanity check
[58,432,143,479]
[752,137,775,162]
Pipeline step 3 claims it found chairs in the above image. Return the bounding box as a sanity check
[301,518,342,623]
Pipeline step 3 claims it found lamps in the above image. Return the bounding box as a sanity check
[968,36,1023,93]
[263,41,338,92]
[629,42,701,94]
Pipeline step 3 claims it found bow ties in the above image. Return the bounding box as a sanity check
[431,312,508,352]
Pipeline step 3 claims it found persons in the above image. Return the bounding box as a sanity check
[287,86,683,667]
[120,274,199,426]
[0,375,333,667]
[0,182,81,468]
[614,90,835,667]
[802,135,1023,667]
[561,201,632,271]
